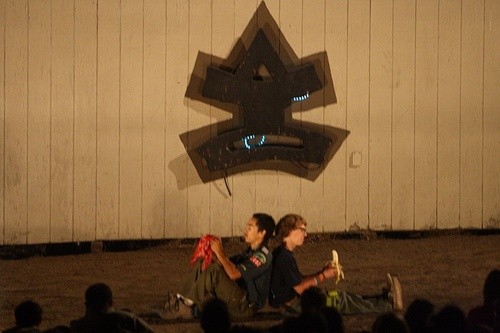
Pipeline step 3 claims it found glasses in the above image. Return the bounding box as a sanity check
[299,227,306,231]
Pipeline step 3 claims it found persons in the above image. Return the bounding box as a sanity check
[7,266,496,332]
[145,213,274,323]
[274,213,403,318]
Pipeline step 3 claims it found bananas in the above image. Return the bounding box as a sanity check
[322,249,344,284]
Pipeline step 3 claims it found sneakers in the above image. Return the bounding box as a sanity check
[150,290,178,313]
[389,276,403,311]
[159,297,192,320]
[386,273,394,297]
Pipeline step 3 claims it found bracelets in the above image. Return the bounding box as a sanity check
[313,271,326,285]
